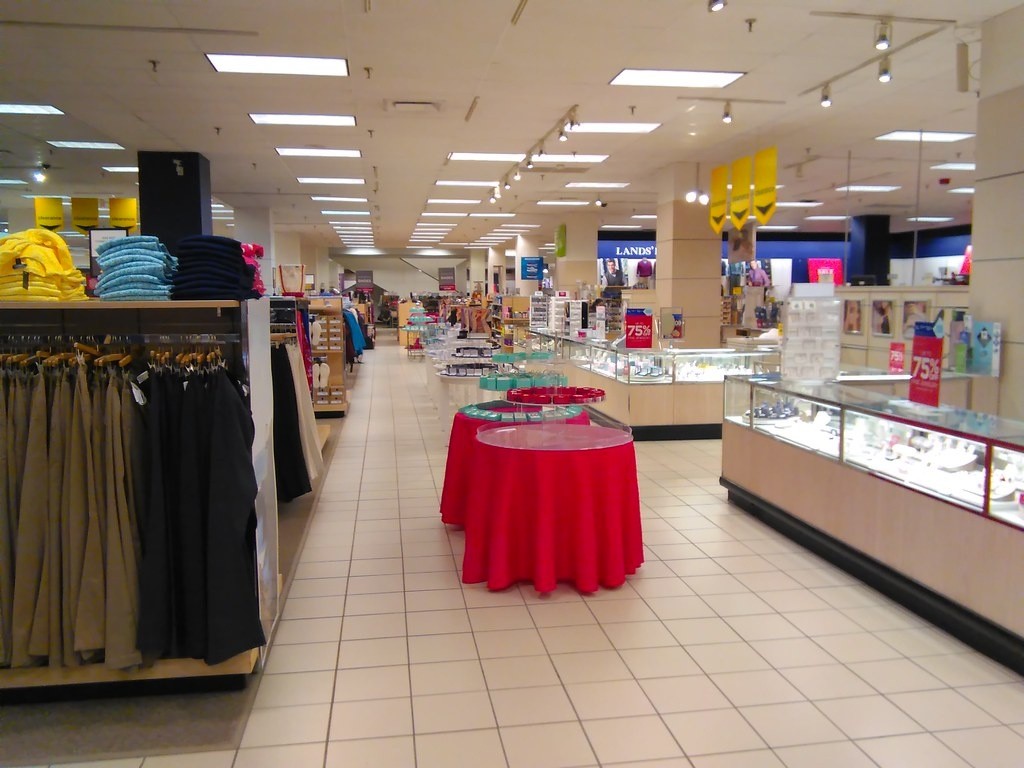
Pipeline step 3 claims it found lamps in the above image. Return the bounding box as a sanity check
[486,103,582,204]
[722,102,733,124]
[872,18,894,84]
[819,82,833,108]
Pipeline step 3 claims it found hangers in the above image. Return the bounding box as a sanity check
[268,321,300,351]
[0,334,232,379]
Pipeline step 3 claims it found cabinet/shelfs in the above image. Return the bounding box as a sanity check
[385,284,530,373]
[308,293,375,419]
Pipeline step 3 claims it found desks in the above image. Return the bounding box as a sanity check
[401,298,646,594]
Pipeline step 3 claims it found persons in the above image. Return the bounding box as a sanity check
[727,257,744,296]
[748,259,769,301]
[636,256,652,289]
[380,306,391,324]
[592,299,607,330]
[602,260,626,299]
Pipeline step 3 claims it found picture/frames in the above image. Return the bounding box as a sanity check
[841,296,933,342]
